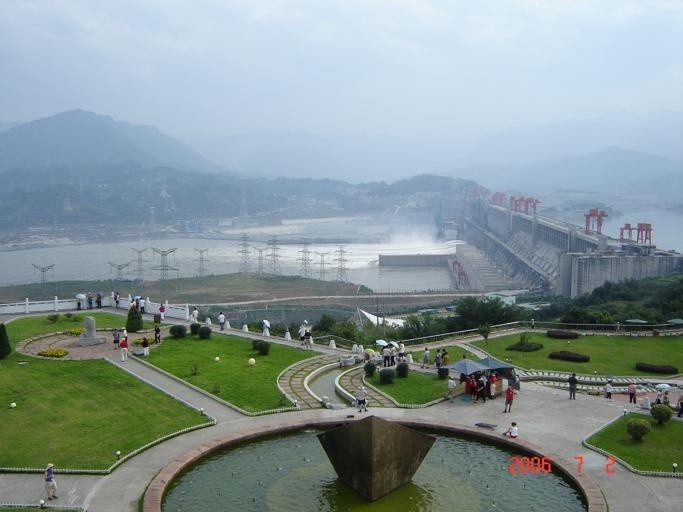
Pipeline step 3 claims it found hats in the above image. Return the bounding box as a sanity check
[357,386,364,389]
[46,464,53,471]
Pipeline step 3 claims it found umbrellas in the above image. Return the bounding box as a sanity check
[263,320,270,328]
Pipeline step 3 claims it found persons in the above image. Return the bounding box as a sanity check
[356,385,369,412]
[655,389,682,417]
[298,319,314,349]
[628,381,638,404]
[363,340,405,367]
[76,291,165,362]
[614,321,625,333]
[603,380,612,399]
[44,463,58,500]
[192,308,198,322]
[421,348,521,438]
[568,373,578,399]
[218,312,225,330]
[527,319,535,329]
[262,320,270,336]
